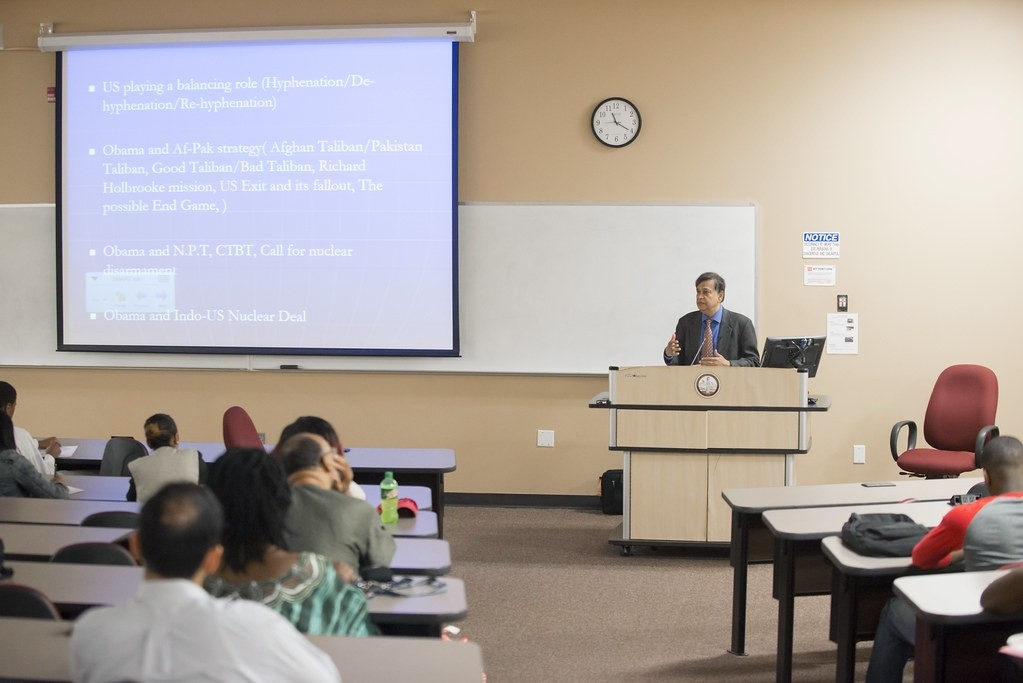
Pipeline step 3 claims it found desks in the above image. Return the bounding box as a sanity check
[762,500,955,683]
[892,568,1013,683]
[721,477,985,656]
[0,437,494,683]
[821,535,918,683]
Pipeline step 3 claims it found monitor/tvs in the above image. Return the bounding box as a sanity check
[761,336,826,378]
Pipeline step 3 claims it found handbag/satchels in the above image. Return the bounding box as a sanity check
[599,469,623,515]
[842,513,929,558]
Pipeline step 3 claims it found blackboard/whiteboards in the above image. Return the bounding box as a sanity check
[0,203,758,377]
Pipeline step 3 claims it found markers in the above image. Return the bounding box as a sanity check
[280,364,302,369]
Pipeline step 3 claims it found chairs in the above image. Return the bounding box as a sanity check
[888,364,1000,481]
[51,542,138,565]
[80,511,139,529]
[0,583,61,621]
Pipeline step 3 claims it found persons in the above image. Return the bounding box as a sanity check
[663,272,760,367]
[271,435,396,583]
[127,414,210,503]
[981,564,1023,614]
[865,435,1023,683]
[0,381,61,474]
[0,411,68,499]
[69,482,342,683]
[202,447,380,636]
[278,416,366,499]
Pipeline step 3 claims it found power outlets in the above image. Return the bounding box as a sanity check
[537,429,555,447]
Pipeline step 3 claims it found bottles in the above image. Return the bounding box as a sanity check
[441,622,468,642]
[379,472,399,527]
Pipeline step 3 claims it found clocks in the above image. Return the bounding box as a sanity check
[591,96,643,148]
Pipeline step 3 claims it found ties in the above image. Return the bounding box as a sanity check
[701,319,713,361]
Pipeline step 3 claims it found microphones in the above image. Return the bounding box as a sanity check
[691,337,706,365]
